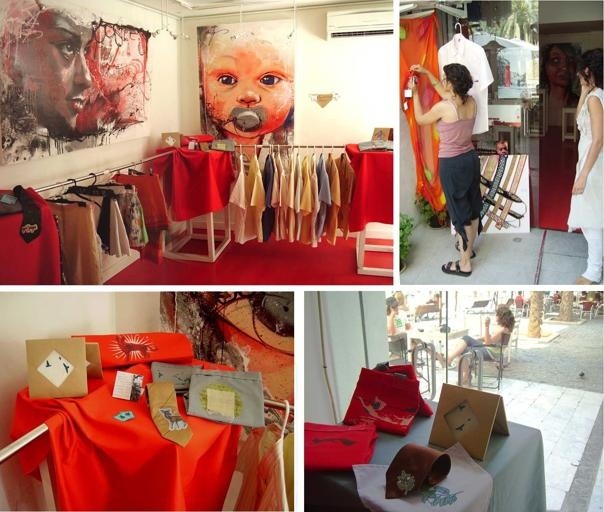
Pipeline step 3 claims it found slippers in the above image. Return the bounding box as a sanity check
[443,260,471,276]
[455,241,475,258]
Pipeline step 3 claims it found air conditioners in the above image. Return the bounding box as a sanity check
[326,9,395,40]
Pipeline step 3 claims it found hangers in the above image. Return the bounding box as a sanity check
[451,21,463,42]
[45,160,152,208]
[237,143,347,167]
[276,399,294,447]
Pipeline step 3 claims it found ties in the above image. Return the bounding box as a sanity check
[386,443,451,498]
[146,383,193,448]
[13,185,41,244]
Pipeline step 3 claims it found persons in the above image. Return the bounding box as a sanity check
[541,43,580,127]
[515,291,525,308]
[387,297,444,362]
[579,291,596,311]
[566,48,604,285]
[435,304,515,386]
[5,10,94,137]
[552,291,560,304]
[496,140,509,155]
[199,26,294,162]
[407,63,482,277]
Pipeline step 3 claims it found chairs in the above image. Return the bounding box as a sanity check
[473,332,510,391]
[515,300,524,316]
[387,333,408,361]
[580,302,594,320]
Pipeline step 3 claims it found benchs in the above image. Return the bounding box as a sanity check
[415,303,440,320]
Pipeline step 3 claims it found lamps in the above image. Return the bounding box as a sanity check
[152,1,296,39]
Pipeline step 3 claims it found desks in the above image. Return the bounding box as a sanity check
[411,328,469,397]
[306,398,547,511]
